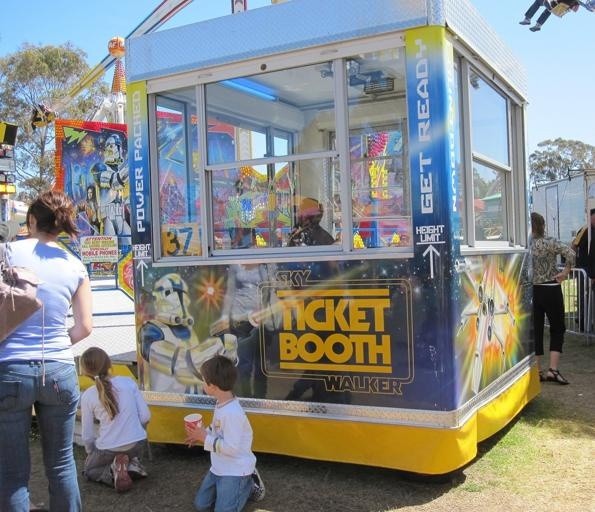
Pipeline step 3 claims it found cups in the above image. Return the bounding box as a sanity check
[183,412,203,430]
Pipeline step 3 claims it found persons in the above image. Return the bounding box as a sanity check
[182,356,266,512]
[78,185,104,238]
[295,198,334,244]
[220,263,279,400]
[226,179,256,249]
[530,212,577,385]
[80,347,151,493]
[570,208,595,333]
[519,0,578,32]
[0,189,95,512]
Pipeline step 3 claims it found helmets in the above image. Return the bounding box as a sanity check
[152,272,191,318]
[295,197,325,224]
[103,132,123,157]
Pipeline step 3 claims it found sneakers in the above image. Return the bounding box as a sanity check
[128,456,149,479]
[111,452,134,495]
[249,467,266,503]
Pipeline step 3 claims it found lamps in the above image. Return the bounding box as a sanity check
[321,58,395,95]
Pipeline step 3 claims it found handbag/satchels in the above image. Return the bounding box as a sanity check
[0,264,43,345]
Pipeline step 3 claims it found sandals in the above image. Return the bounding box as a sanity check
[539,370,546,381]
[547,368,571,386]
